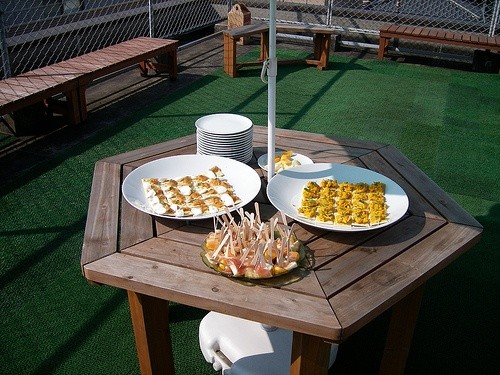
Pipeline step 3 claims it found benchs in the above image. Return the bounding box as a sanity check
[0,37,179,139]
[223,24,269,78]
[376,23,500,60]
[214,19,336,70]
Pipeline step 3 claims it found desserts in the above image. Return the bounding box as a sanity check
[141,165,242,216]
[296,178,388,227]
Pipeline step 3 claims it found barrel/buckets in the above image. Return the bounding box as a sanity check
[200,310,337,375]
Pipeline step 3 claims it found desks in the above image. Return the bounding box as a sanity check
[80,124,483,375]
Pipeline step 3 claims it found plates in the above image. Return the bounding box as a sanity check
[195,113,254,163]
[257,151,314,173]
[199,228,307,281]
[266,162,410,232]
[122,154,261,220]
